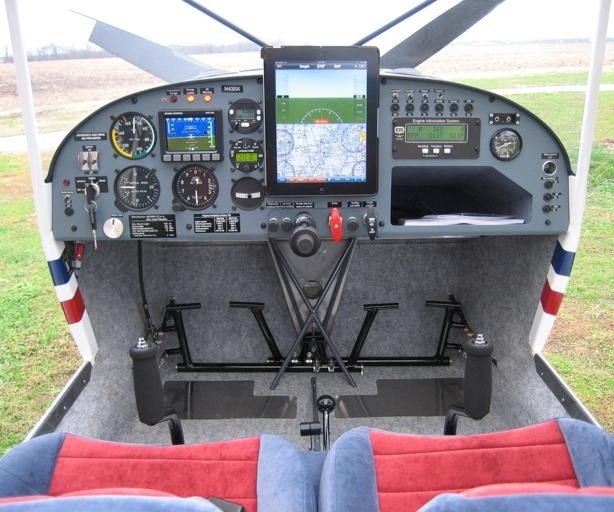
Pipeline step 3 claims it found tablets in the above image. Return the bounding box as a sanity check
[261,45,379,196]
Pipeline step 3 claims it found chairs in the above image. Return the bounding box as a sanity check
[317,416,614,510]
[2,429,314,510]
